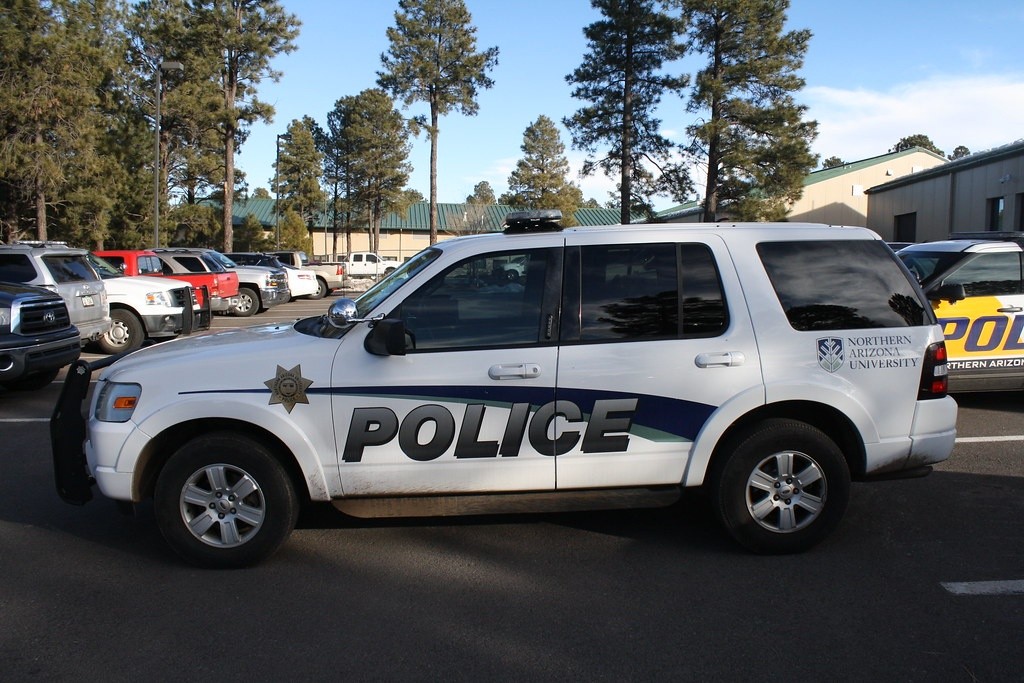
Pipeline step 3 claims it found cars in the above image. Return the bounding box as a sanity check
[0,280,83,390]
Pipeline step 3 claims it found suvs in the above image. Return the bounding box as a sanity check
[143,247,321,318]
[886,230,1024,394]
[16,239,213,355]
[0,244,112,350]
[82,206,977,573]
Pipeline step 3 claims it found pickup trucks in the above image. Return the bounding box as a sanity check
[260,249,349,299]
[318,252,406,280]
[82,247,243,325]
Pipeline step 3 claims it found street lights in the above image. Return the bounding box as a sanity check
[152,61,187,245]
[275,132,292,250]
